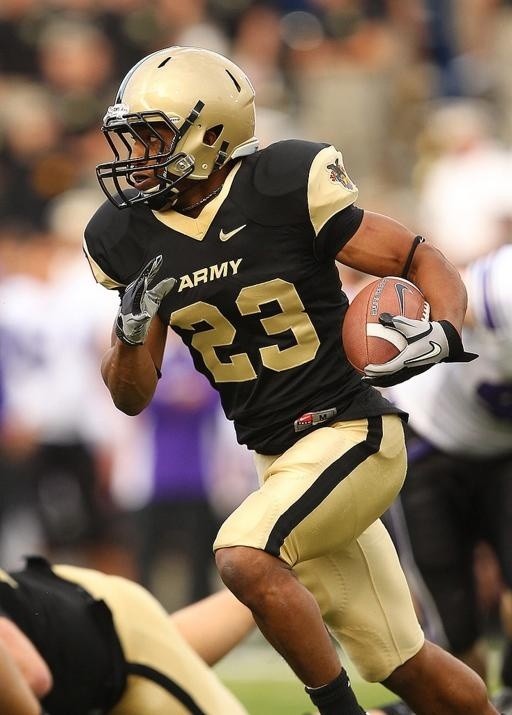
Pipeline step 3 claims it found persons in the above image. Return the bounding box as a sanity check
[1,0,510,661]
[0,554,258,715]
[358,237,511,714]
[78,42,501,715]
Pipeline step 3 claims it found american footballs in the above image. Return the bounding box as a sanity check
[343,276,430,374]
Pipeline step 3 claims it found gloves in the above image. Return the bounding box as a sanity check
[359,313,478,387]
[114,255,175,346]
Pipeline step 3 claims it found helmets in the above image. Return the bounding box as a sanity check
[95,45,258,211]
